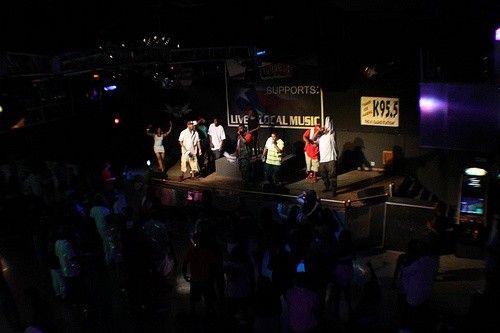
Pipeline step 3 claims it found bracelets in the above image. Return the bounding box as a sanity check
[146,129,150,130]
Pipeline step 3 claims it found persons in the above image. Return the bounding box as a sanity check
[196,117,208,152]
[240,108,260,154]
[207,118,226,159]
[235,124,250,186]
[0,163,353,333]
[390,238,439,319]
[260,131,285,184]
[342,252,383,333]
[424,200,447,267]
[147,121,173,171]
[287,272,320,333]
[178,121,202,182]
[302,111,338,198]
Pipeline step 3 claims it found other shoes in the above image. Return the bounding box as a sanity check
[312,174,317,182]
[306,174,309,181]
[322,186,329,192]
[331,189,336,196]
